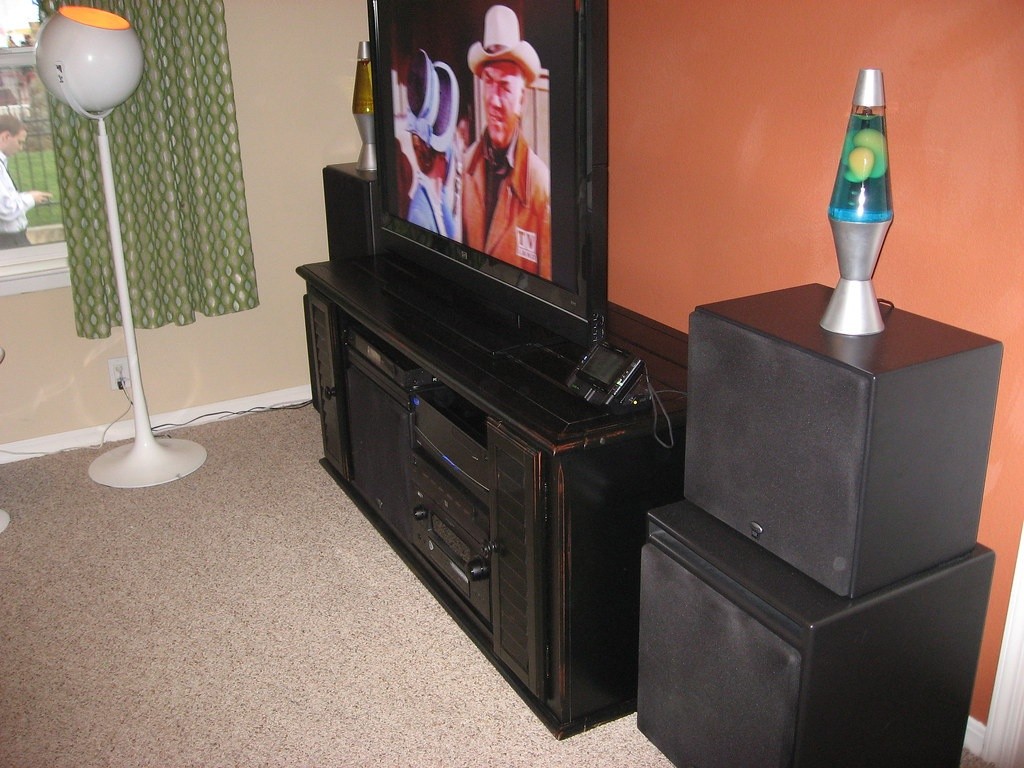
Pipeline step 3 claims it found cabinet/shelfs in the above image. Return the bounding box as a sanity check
[107,356,132,391]
[295,261,689,742]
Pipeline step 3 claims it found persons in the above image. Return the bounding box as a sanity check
[400,3,553,280]
[0,113,52,249]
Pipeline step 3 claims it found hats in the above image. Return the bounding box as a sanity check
[468,4,541,88]
[403,48,459,153]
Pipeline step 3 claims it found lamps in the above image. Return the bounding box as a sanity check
[34,4,208,489]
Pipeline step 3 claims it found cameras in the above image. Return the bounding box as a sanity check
[568,340,646,412]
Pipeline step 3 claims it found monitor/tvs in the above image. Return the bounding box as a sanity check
[365,0,608,374]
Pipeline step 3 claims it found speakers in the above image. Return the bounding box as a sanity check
[685,283,1005,599]
[635,502,998,768]
[323,162,386,280]
